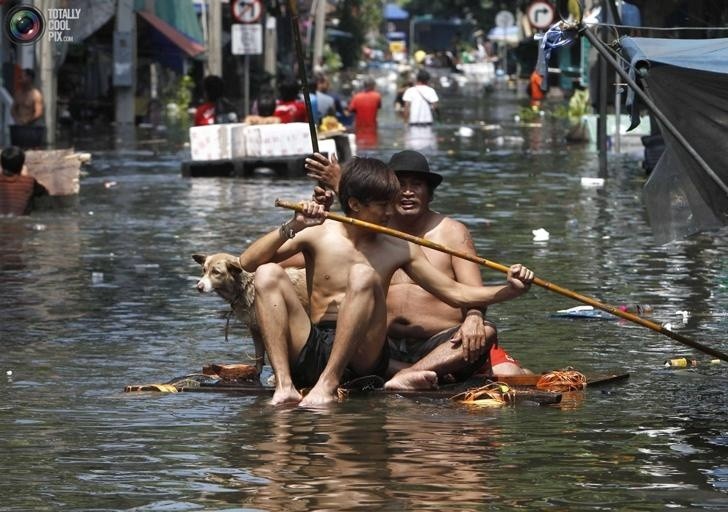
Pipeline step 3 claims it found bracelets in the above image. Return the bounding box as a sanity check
[279,222,295,239]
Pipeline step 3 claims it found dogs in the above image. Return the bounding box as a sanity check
[192,253,310,373]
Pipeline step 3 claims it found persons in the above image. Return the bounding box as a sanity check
[11,68,46,150]
[531,67,545,100]
[395,45,481,127]
[239,156,534,407]
[348,77,382,132]
[383,150,497,391]
[0,146,49,216]
[243,74,355,132]
[304,152,340,212]
[194,76,240,126]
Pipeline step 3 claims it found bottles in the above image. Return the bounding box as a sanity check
[620,304,654,314]
[664,356,721,372]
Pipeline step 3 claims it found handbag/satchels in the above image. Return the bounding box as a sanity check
[430,106,439,120]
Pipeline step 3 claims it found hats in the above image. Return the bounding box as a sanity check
[388,151,443,189]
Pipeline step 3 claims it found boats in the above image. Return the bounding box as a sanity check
[181,134,352,182]
[121,362,632,410]
